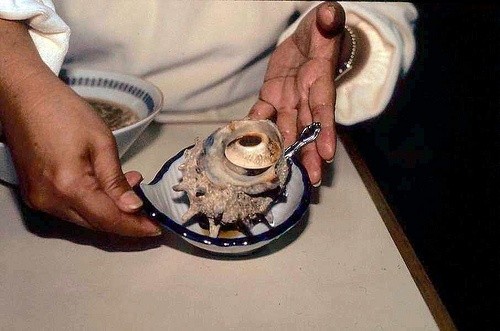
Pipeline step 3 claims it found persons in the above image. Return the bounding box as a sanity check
[0,1,419,236]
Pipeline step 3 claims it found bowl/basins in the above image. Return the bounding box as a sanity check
[0,69,163,184]
[134,140,312,256]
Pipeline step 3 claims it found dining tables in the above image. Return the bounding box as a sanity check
[0,123,457,331]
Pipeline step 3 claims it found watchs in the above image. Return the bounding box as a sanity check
[334,25,357,82]
